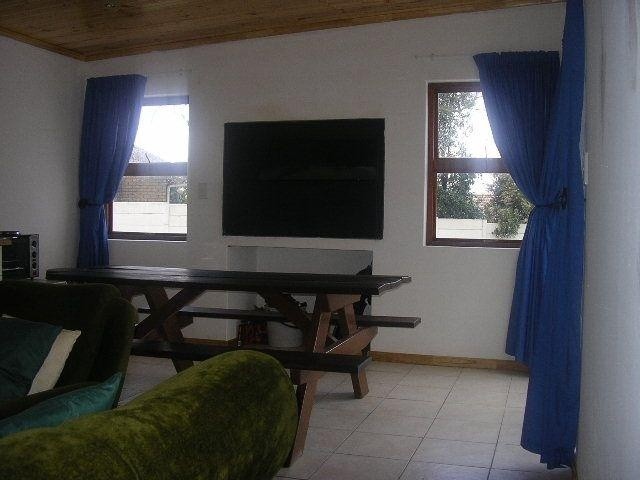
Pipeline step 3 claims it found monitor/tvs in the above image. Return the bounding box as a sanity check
[222,117,386,241]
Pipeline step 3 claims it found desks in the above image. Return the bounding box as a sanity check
[44,264,413,469]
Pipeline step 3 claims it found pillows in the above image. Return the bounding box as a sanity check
[1,371,127,434]
[1,313,83,395]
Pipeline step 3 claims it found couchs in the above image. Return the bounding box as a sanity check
[0,348,301,475]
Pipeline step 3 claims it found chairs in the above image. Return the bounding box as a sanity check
[1,277,140,416]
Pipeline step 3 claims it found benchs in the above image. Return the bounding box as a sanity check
[133,304,424,400]
[133,337,374,470]
